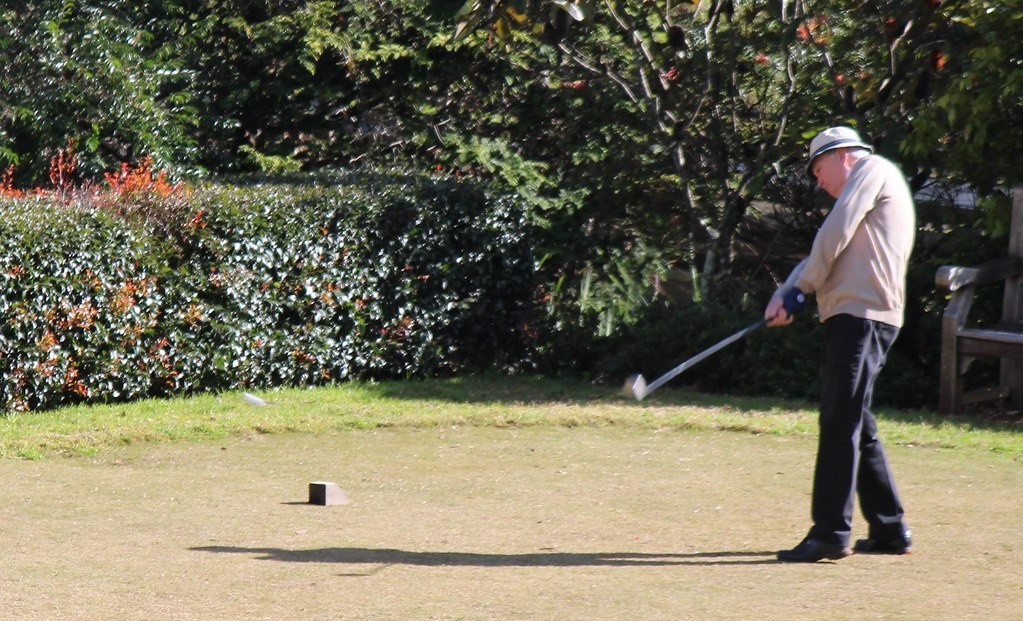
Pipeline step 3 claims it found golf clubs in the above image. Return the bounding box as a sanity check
[621,294,807,400]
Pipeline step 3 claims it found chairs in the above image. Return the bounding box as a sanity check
[929,187,1023,415]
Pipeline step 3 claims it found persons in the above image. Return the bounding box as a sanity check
[761,125,917,563]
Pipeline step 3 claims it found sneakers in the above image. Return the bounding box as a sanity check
[853,530,911,556]
[776,534,852,563]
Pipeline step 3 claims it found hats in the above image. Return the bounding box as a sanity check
[804,126,875,179]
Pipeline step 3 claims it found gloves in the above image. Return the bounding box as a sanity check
[783,286,808,320]
